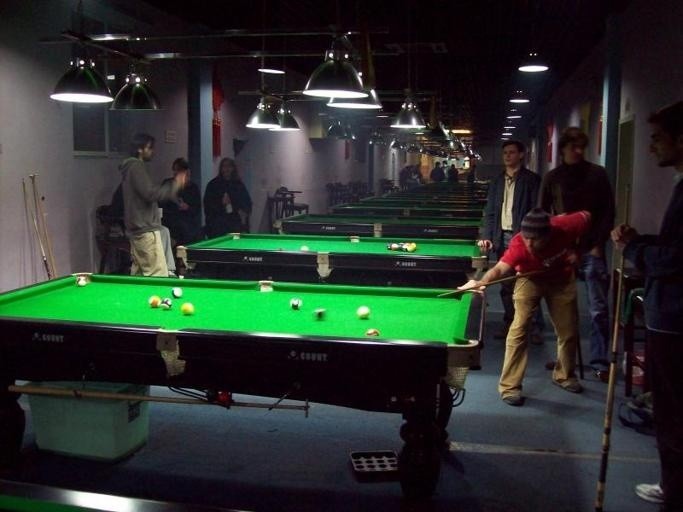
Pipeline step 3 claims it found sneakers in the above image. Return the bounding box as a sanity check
[634,482,666,504]
[552,380,585,393]
[503,396,523,407]
[493,321,617,384]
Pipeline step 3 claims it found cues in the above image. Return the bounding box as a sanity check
[22,172,58,279]
[6,385,310,412]
[437,266,565,296]
[592,182,632,511]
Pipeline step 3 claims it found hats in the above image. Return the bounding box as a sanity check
[520,207,553,239]
[556,126,590,156]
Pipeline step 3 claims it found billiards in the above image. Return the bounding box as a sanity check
[182,302,193,315]
[291,298,301,308]
[173,286,182,297]
[367,329,378,337]
[301,245,308,251]
[387,242,417,253]
[162,298,171,309]
[316,308,327,320]
[150,295,160,307]
[357,306,370,320]
[77,275,88,287]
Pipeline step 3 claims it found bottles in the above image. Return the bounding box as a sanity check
[223,193,233,216]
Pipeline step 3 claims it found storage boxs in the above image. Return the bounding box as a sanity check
[24,381,149,461]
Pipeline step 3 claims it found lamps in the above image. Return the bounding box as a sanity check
[49,3,117,105]
[108,26,166,112]
[302,27,426,144]
[493,3,551,141]
[390,121,481,162]
[246,56,300,132]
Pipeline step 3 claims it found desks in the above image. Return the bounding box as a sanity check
[175,180,493,370]
[0,271,485,511]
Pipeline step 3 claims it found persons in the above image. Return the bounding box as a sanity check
[101,183,131,275]
[398,161,477,189]
[610,99,683,511]
[476,141,545,346]
[535,128,616,383]
[456,207,591,406]
[117,134,188,277]
[204,158,250,239]
[158,158,199,242]
